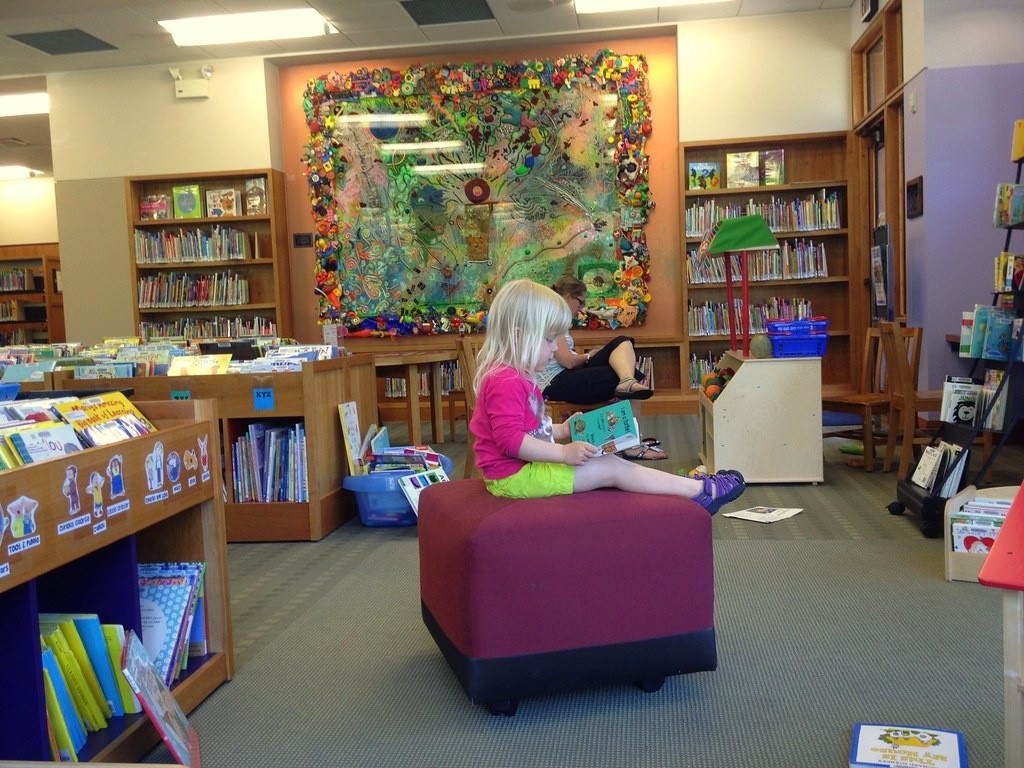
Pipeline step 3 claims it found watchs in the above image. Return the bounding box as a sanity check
[584,354,591,364]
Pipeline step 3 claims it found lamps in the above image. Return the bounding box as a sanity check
[169,64,217,101]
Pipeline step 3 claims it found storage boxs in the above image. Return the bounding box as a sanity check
[943,485,1019,583]
[767,317,831,357]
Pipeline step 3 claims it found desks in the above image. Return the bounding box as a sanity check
[978,484,1024,768]
[368,349,458,446]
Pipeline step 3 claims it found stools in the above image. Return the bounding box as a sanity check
[417,477,720,715]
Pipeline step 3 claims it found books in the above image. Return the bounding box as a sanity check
[911,440,968,499]
[689,148,785,191]
[222,424,308,503]
[689,350,726,390]
[848,722,967,768]
[940,181,1024,432]
[686,189,841,336]
[951,498,1013,555]
[385,359,463,398]
[39,561,207,768]
[0,337,353,382]
[337,401,450,518]
[635,356,655,390]
[134,178,277,339]
[0,269,36,346]
[0,390,160,471]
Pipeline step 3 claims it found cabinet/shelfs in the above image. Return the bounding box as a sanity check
[687,128,866,396]
[53,352,375,543]
[0,242,68,346]
[123,168,291,341]
[698,346,823,485]
[885,118,1024,540]
[0,394,236,768]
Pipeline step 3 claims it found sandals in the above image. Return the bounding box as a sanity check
[684,469,745,516]
[614,378,653,399]
[623,438,668,460]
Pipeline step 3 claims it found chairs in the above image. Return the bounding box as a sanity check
[456,330,605,467]
[822,322,996,478]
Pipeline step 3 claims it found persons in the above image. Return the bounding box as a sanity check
[535,275,668,461]
[469,278,745,515]
